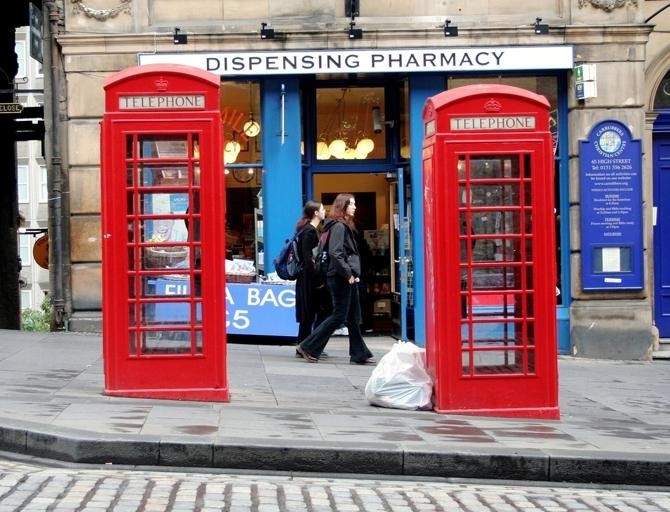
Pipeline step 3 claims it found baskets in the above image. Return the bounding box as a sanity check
[146,248,186,268]
[225,274,253,284]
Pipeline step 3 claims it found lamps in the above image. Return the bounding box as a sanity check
[370,105,395,135]
[173,26,188,46]
[348,22,363,40]
[260,21,275,40]
[315,89,375,162]
[534,19,549,34]
[444,21,458,37]
[221,78,262,163]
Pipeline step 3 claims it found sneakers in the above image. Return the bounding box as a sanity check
[295,343,328,363]
[350,356,377,365]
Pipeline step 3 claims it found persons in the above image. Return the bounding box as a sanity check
[296,192,377,366]
[295,201,328,360]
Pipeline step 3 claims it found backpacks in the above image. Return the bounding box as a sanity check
[312,230,331,274]
[273,235,302,281]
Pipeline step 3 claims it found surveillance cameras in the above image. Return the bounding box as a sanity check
[371,106,383,135]
